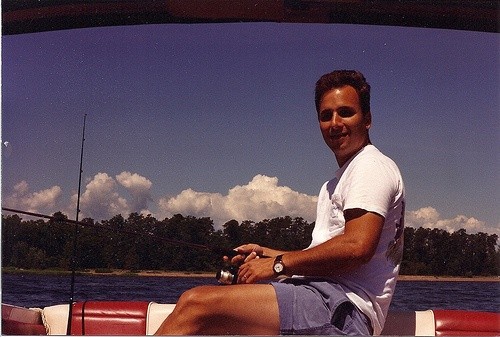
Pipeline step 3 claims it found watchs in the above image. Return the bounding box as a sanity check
[273,254,286,276]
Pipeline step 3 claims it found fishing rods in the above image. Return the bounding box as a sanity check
[66,111,86,336]
[1,190,273,286]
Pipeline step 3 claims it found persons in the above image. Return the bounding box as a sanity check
[155,69,406,336]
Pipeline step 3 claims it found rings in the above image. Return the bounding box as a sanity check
[243,274,248,281]
